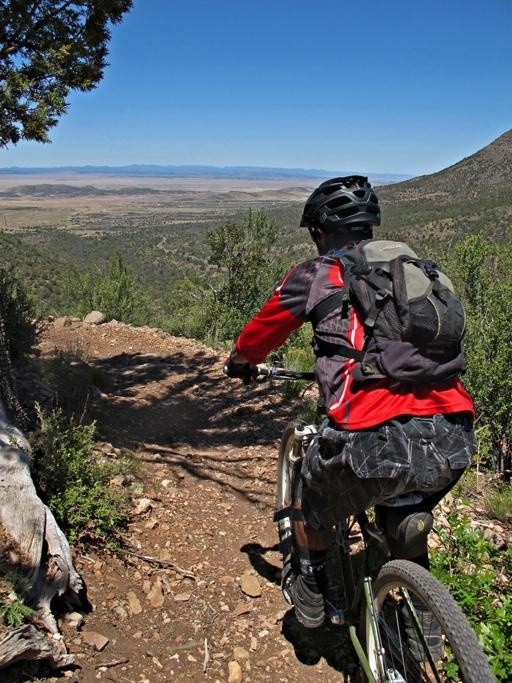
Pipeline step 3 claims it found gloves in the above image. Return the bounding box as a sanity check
[224,355,258,384]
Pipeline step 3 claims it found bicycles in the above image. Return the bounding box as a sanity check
[223,365,494,682]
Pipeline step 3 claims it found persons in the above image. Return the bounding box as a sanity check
[224,175,478,662]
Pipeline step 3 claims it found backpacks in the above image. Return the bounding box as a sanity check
[326,239,467,388]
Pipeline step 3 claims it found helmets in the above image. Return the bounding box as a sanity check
[299,175,381,233]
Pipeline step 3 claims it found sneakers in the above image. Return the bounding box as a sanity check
[281,550,326,629]
[399,600,444,662]
